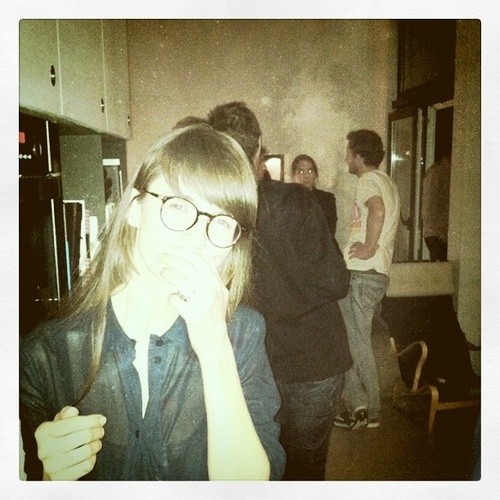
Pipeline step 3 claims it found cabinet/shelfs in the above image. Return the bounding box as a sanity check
[19,19,133,139]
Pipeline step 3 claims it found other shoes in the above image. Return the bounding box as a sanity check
[333,412,380,428]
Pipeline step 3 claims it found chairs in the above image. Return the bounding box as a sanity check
[385,259,480,436]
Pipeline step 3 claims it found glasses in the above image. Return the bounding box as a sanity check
[294,168,315,176]
[141,188,248,249]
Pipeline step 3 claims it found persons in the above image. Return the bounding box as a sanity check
[19,124,288,482]
[203,99,352,481]
[329,127,401,431]
[288,153,337,243]
